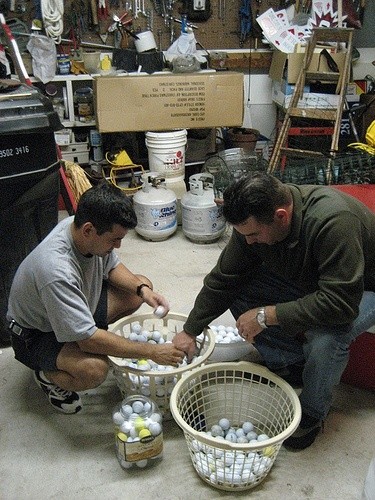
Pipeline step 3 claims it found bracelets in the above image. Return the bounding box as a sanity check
[136,283,153,298]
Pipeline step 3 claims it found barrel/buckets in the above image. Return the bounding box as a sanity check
[145,129,188,178]
[225,128,260,151]
[162,175,188,225]
[185,129,212,162]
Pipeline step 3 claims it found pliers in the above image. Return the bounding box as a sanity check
[66,26,76,53]
[98,0,108,16]
[107,12,133,33]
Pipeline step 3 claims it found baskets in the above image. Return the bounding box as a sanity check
[106,311,215,419]
[169,361,301,492]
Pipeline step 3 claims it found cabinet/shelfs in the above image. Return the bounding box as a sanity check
[29,68,216,166]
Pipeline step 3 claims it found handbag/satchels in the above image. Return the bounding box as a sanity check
[27,34,57,84]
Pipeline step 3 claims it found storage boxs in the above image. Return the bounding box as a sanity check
[0,81,65,348]
[93,71,245,132]
[269,50,366,108]
[61,137,90,164]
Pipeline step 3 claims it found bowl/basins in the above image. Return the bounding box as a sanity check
[198,320,252,362]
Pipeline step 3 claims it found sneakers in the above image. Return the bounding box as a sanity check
[242,363,303,388]
[33,370,82,414]
[283,411,329,451]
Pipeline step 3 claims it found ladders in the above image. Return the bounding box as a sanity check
[266,27,355,185]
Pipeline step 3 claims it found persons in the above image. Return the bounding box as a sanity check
[172,172,374,453]
[6,184,186,414]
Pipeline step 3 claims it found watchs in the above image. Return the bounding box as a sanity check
[256,306,269,329]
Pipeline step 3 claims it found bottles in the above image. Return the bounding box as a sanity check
[112,395,163,473]
[74,87,94,121]
[56,49,111,75]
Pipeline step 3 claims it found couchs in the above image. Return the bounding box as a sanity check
[331,184,375,391]
